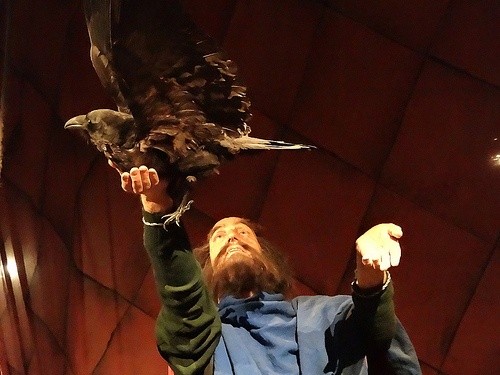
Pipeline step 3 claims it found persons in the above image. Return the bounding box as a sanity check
[106,156,424,375]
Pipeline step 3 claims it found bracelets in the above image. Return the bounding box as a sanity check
[350,269,393,296]
